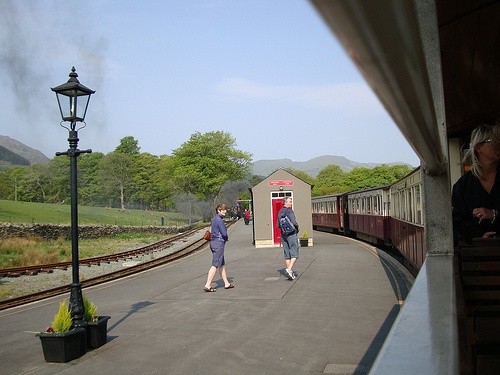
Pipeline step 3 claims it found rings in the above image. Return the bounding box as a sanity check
[478,213,482,218]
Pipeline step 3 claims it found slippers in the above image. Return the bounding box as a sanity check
[224,283,235,289]
[203,287,216,292]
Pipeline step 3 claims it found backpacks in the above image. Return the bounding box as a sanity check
[279,209,296,236]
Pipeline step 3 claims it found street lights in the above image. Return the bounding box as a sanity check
[49,65,96,328]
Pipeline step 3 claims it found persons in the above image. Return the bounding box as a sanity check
[277,197,300,280]
[452,124,500,375]
[203,203,234,291]
[242,209,250,225]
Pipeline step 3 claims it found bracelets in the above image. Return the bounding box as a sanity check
[490,209,495,224]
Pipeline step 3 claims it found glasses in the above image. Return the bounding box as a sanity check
[482,137,498,143]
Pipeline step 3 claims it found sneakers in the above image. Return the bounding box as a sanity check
[283,267,296,280]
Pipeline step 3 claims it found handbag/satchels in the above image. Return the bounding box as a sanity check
[203,230,212,240]
